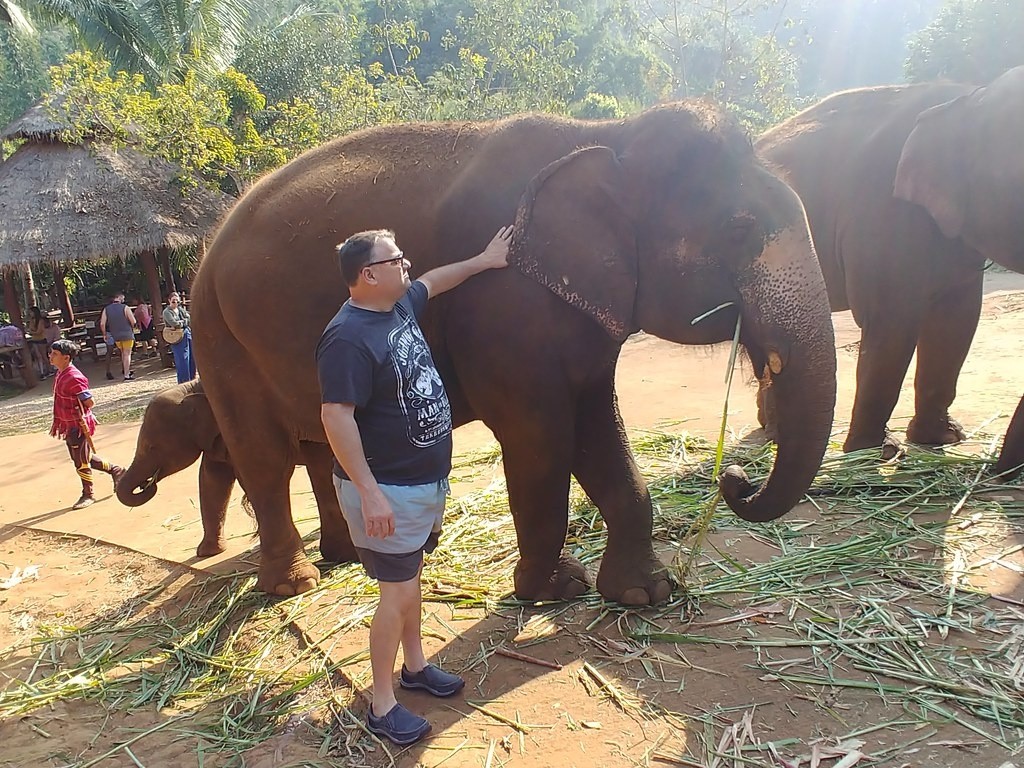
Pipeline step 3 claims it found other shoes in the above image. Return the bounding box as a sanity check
[399,662,466,697]
[40,374,46,381]
[113,467,128,493]
[138,353,149,360]
[366,700,432,746]
[149,352,157,357]
[72,497,97,510]
[124,377,136,382]
[128,373,134,378]
[106,372,114,380]
[46,371,55,378]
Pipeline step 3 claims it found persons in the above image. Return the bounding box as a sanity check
[49,340,126,511]
[133,295,157,361]
[28,306,55,381]
[314,224,516,747]
[100,291,137,383]
[42,317,61,359]
[163,291,196,384]
[102,296,128,380]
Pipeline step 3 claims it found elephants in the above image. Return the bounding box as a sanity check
[114,373,357,568]
[747,60,1022,487]
[187,97,841,611]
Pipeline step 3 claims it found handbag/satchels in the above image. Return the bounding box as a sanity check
[162,326,185,345]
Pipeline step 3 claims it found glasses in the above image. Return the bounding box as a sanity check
[360,251,404,274]
[171,301,181,304]
